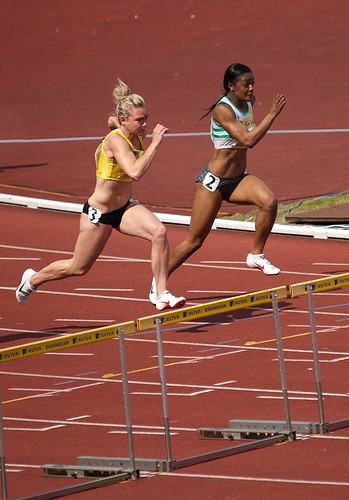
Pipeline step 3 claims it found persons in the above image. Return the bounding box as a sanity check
[16,76,186,310]
[147,62,287,305]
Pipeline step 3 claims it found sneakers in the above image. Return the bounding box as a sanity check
[148,276,158,305]
[16,268,37,305]
[156,290,186,311]
[246,253,280,276]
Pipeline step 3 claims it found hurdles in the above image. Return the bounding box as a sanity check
[0,272,349,499]
